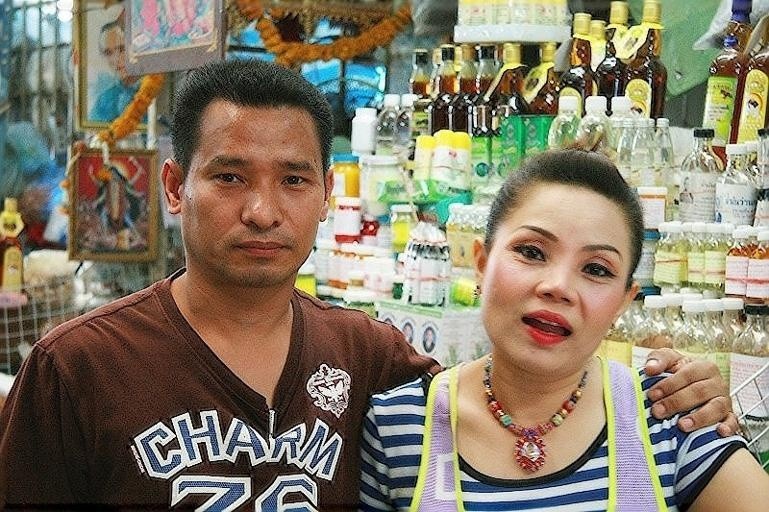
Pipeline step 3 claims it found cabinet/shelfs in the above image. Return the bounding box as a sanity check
[0,197,23,291]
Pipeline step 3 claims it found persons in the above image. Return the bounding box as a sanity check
[0,59,740,512]
[358,147,769,511]
[88,22,149,123]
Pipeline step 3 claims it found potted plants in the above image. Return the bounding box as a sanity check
[70,0,170,136]
[64,145,159,264]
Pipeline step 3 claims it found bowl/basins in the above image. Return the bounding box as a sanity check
[481,352,590,473]
